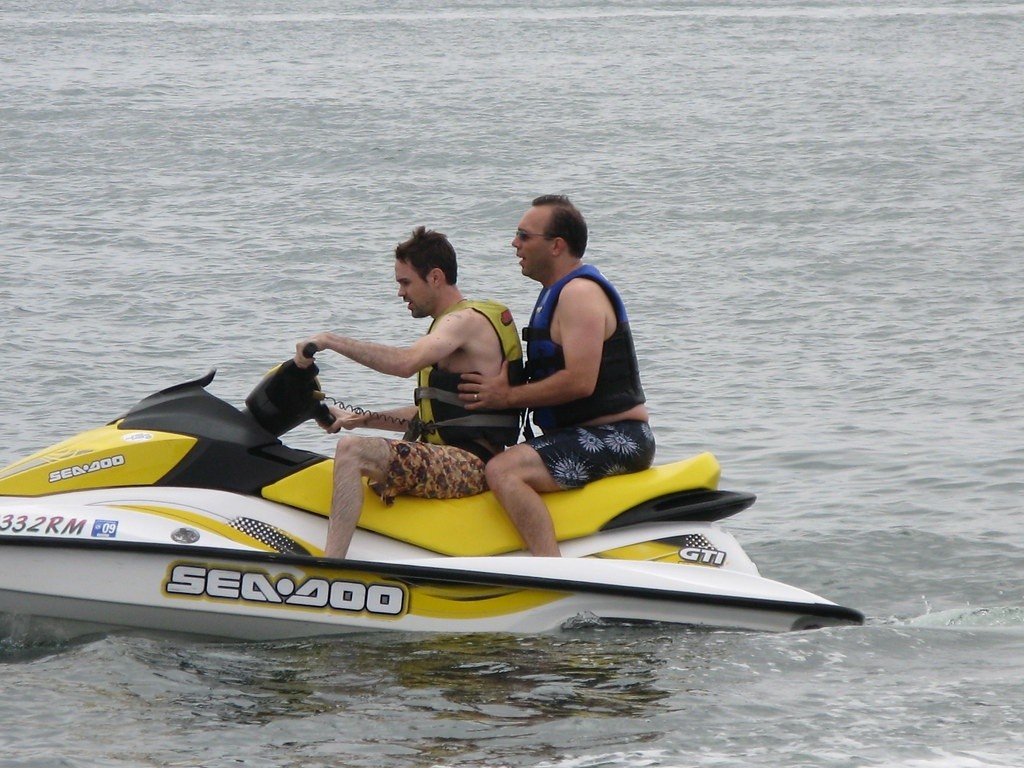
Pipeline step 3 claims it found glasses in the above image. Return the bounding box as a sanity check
[516,231,546,240]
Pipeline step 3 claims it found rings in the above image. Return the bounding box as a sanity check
[474,395,478,401]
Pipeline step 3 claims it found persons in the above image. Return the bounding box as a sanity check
[456,194,655,557]
[292,226,523,560]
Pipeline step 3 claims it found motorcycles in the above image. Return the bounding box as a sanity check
[0,342,866,642]
